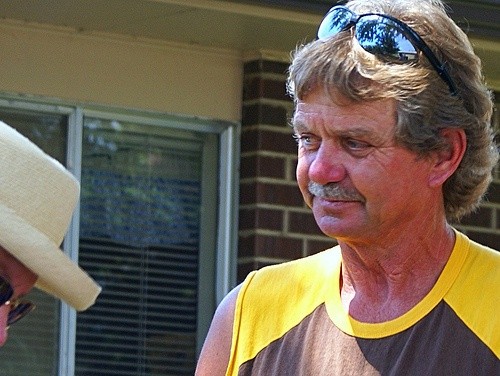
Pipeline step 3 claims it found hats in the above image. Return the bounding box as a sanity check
[0,120,104,312]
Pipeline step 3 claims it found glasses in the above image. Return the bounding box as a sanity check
[0,276,34,325]
[316,5,460,98]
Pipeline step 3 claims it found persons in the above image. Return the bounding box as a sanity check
[195,0,500,376]
[0,121,102,348]
